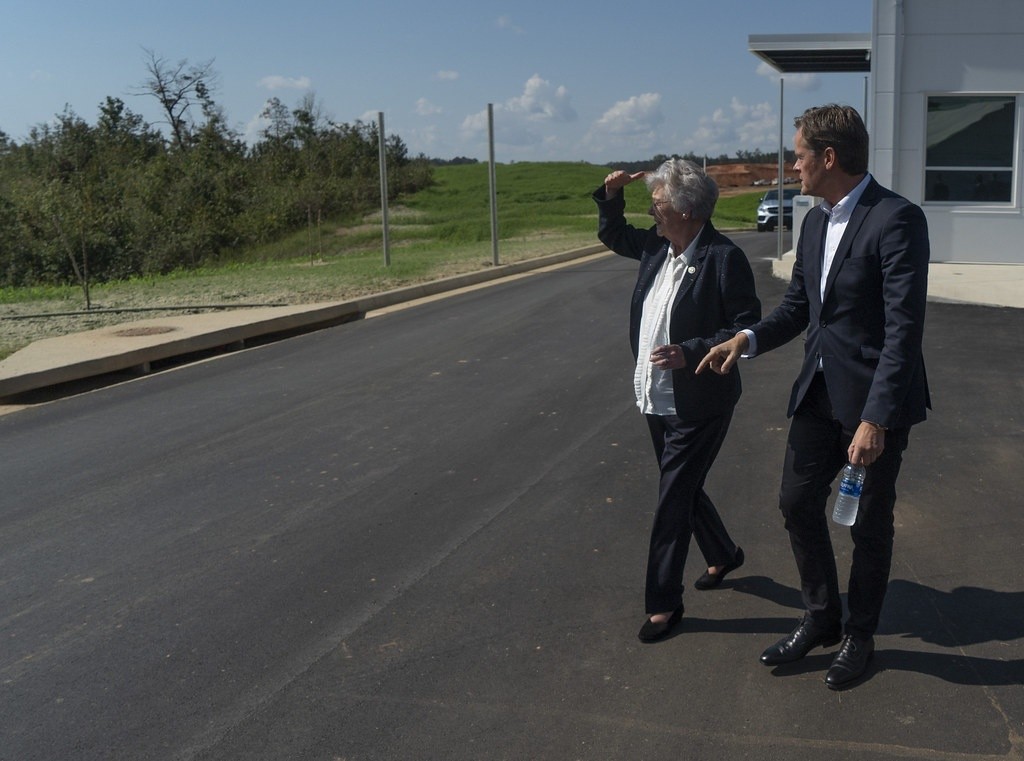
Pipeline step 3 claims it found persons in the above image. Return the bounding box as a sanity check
[694,106,931,691]
[593,160,761,642]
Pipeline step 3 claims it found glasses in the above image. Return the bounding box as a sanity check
[653,199,671,209]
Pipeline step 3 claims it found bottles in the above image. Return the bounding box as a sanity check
[833,464,867,524]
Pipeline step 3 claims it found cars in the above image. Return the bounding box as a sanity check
[756,188,803,232]
[753,176,797,185]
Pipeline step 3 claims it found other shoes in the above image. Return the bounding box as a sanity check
[638,601,684,641]
[695,547,744,590]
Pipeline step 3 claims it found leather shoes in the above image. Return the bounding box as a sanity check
[759,614,841,665]
[825,635,875,688]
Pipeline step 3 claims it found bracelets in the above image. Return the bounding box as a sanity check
[877,425,886,430]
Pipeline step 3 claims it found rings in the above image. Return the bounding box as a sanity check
[665,359,667,364]
